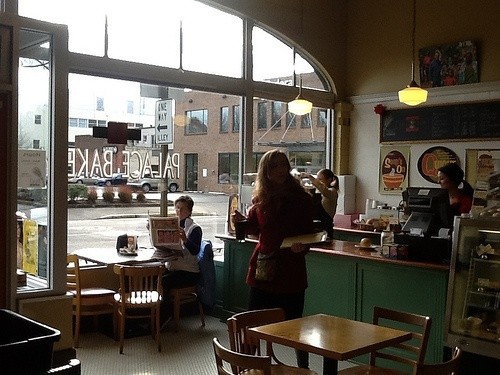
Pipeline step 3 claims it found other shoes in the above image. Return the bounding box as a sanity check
[158,314,171,330]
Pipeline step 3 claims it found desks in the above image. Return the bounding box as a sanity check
[76,247,158,339]
[248,314,412,375]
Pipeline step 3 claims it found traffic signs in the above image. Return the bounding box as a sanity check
[155,98,175,145]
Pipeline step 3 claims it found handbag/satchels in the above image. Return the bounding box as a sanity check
[255,254,276,282]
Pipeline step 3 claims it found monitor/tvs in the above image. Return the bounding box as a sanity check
[400,187,452,237]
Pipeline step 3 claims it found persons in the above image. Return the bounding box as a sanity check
[147,194,202,326]
[232,149,316,369]
[418,47,478,88]
[437,163,474,213]
[299,168,339,238]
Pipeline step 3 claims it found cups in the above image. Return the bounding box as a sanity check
[359,198,378,222]
[234,217,248,243]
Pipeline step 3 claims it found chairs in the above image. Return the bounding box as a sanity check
[62,241,209,353]
[212,306,462,375]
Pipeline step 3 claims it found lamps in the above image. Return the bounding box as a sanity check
[289,0,313,116]
[398,0,428,106]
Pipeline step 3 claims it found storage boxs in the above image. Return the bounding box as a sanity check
[0,309,62,375]
[334,175,361,229]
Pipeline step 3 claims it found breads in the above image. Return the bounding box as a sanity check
[359,237,371,246]
[479,208,500,216]
[366,219,374,224]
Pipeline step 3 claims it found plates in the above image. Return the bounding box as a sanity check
[354,244,379,248]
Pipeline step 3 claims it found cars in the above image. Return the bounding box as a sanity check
[95,173,130,186]
[127,171,180,193]
[69,173,100,185]
